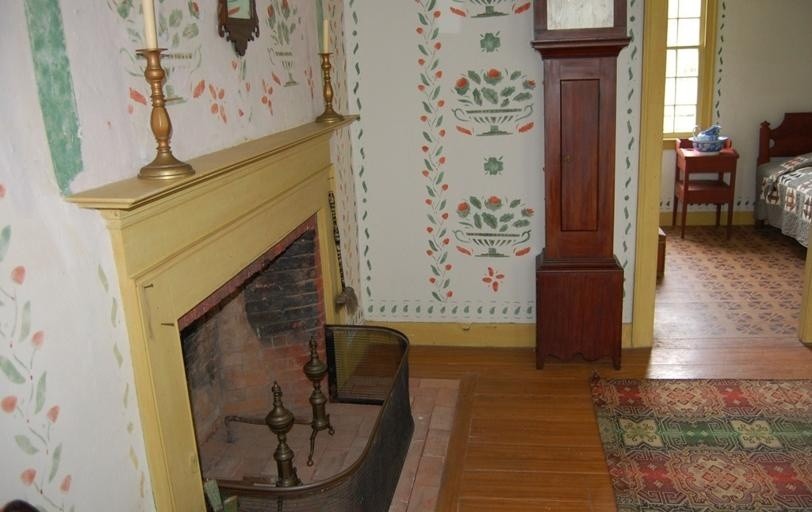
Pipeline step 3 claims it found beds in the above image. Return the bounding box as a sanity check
[755,112,812,249]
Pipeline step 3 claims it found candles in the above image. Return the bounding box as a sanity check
[321,15,330,54]
[140,0,159,50]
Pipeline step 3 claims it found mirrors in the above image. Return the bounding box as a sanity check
[217,0,260,56]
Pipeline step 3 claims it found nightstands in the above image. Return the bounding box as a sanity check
[674,137,740,240]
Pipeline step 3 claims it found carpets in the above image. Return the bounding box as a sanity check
[586,376,812,512]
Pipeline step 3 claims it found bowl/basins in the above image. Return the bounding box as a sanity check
[688,136,728,154]
